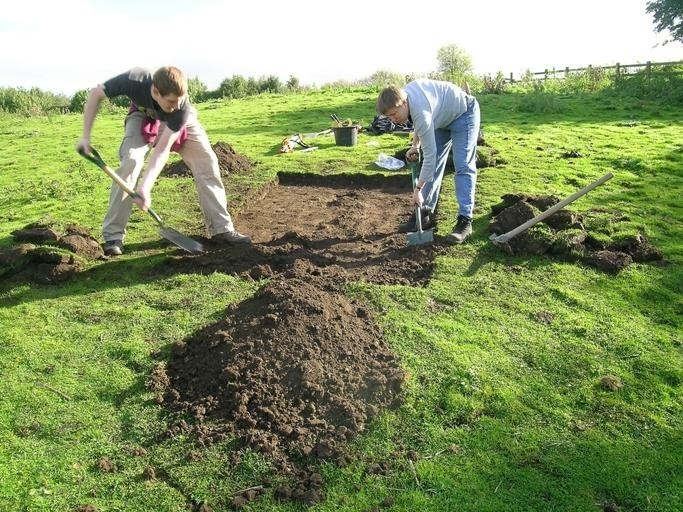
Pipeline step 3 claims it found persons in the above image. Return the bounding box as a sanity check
[76,66,251,256]
[375,78,480,245]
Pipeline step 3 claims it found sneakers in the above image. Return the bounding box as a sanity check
[105,239,122,255]
[399,211,431,231]
[211,231,251,244]
[445,215,472,244]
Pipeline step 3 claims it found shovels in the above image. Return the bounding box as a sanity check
[79,143,205,254]
[406,152,434,247]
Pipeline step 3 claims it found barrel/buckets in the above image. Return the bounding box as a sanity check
[333,125,358,146]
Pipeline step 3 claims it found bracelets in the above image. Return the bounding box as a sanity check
[416,186,421,191]
